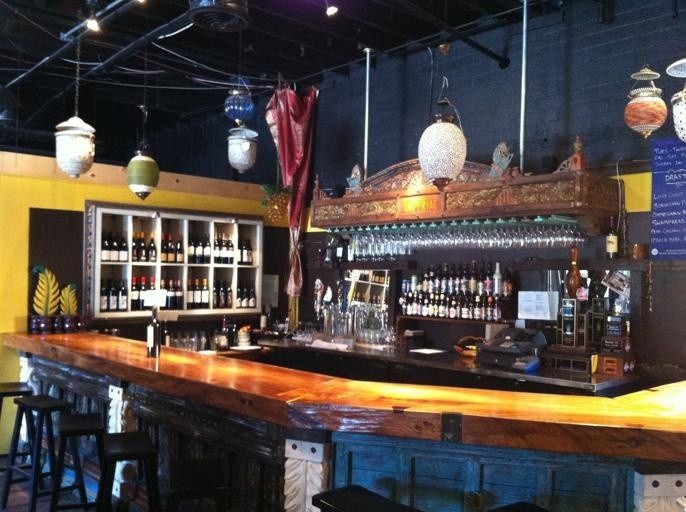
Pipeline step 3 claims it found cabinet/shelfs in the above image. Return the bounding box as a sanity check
[83,201,264,321]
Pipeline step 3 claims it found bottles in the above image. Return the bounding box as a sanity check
[176,279,183,309]
[248,247,252,265]
[221,232,228,263]
[242,280,249,308]
[167,232,177,262]
[236,279,244,308]
[119,232,129,261]
[166,278,176,309]
[219,279,227,308]
[238,240,243,265]
[139,232,147,261]
[213,280,218,308]
[194,278,201,308]
[202,278,208,308]
[101,277,107,311]
[188,278,194,309]
[132,276,139,311]
[147,304,161,357]
[242,240,248,264]
[148,232,156,262]
[118,280,128,310]
[161,279,168,310]
[214,232,220,263]
[110,280,118,311]
[203,241,210,263]
[195,241,204,263]
[139,276,149,310]
[399,259,512,321]
[176,233,184,263]
[101,231,110,261]
[133,232,138,261]
[110,231,119,261]
[149,277,157,310]
[228,233,233,264]
[226,280,232,308]
[189,240,195,262]
[219,317,229,352]
[248,280,256,308]
[161,231,168,262]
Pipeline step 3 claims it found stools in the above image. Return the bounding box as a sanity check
[49,412,112,512]
[313,485,424,512]
[0,381,45,493]
[173,469,228,510]
[1,395,86,510]
[99,432,161,512]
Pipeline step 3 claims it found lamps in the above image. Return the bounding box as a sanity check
[624,65,667,140]
[665,60,686,142]
[417,44,466,192]
[128,43,160,201]
[54,39,97,179]
[225,121,258,174]
[224,33,256,127]
